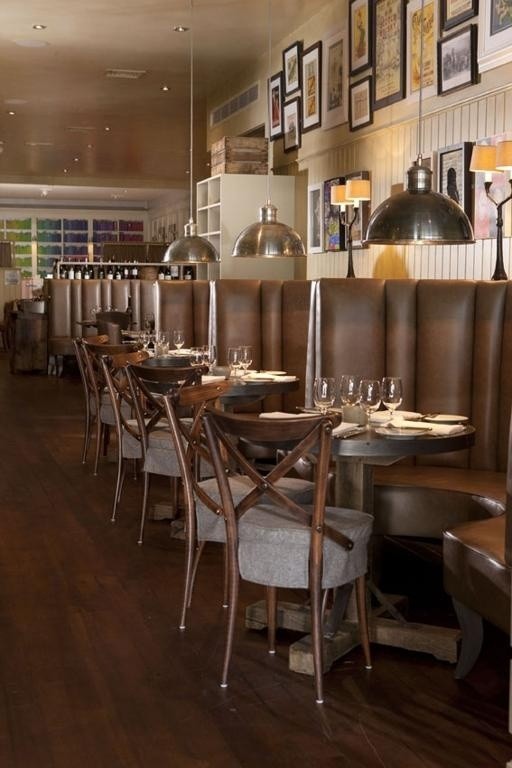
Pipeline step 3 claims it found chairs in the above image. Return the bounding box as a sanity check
[72,337,117,465]
[123,359,239,545]
[160,380,316,655]
[81,336,163,480]
[201,405,375,704]
[100,350,195,523]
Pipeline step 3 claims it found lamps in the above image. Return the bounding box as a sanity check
[162,223,223,266]
[468,140,512,282]
[330,178,370,278]
[230,200,308,260]
[365,155,477,247]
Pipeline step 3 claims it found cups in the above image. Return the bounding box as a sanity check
[158,330,169,348]
[173,330,184,353]
[312,377,337,413]
[204,346,216,367]
[139,331,150,352]
[152,330,157,350]
[239,346,253,373]
[380,377,403,421]
[358,379,380,425]
[228,348,242,379]
[189,347,204,366]
[338,375,362,406]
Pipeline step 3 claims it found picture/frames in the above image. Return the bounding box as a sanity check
[267,71,284,142]
[322,28,349,134]
[301,40,323,135]
[344,171,370,250]
[348,0,373,79]
[483,0,512,59]
[283,42,303,95]
[436,141,472,225]
[281,97,302,155]
[351,75,374,133]
[306,182,324,254]
[436,23,478,98]
[324,176,345,252]
[407,1,440,107]
[370,1,407,113]
[440,0,480,35]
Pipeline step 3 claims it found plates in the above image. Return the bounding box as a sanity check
[374,423,432,439]
[266,371,287,375]
[421,413,469,424]
[242,373,275,385]
[369,410,421,423]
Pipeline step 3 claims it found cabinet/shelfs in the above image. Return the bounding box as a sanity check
[56,261,199,280]
[195,173,296,280]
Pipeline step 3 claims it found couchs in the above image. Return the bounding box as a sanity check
[317,277,512,681]
[50,278,213,379]
[212,278,316,475]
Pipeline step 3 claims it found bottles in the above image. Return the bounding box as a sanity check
[158,266,164,280]
[132,258,138,279]
[185,265,194,281]
[165,266,172,281]
[124,259,130,279]
[107,258,113,279]
[68,265,74,280]
[84,257,90,279]
[75,259,82,279]
[99,257,105,279]
[115,266,121,279]
